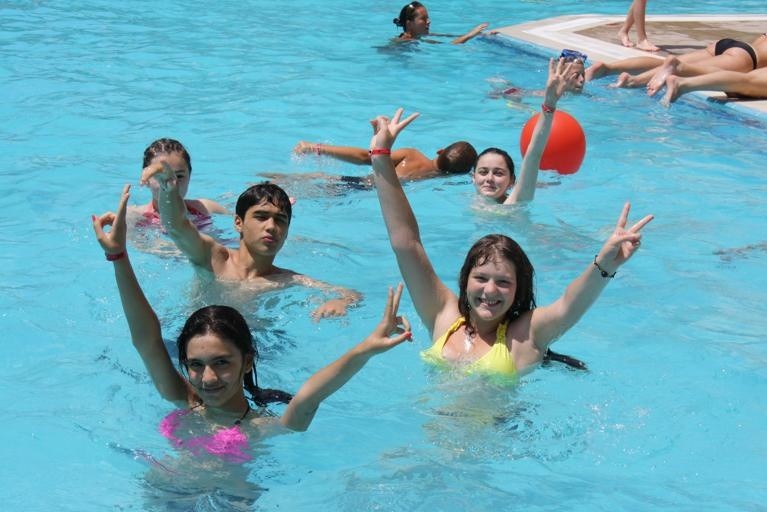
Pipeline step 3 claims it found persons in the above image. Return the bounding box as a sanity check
[617,0,662,51]
[471,53,582,219]
[482,50,608,109]
[393,0,501,45]
[369,107,654,383]
[135,152,365,325]
[118,138,233,228]
[584,30,766,89]
[91,183,413,469]
[642,54,766,108]
[258,140,478,201]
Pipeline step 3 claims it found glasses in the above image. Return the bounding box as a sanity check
[558,49,588,64]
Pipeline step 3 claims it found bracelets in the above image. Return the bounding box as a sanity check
[542,103,556,114]
[368,147,392,157]
[104,249,129,263]
[593,254,618,280]
